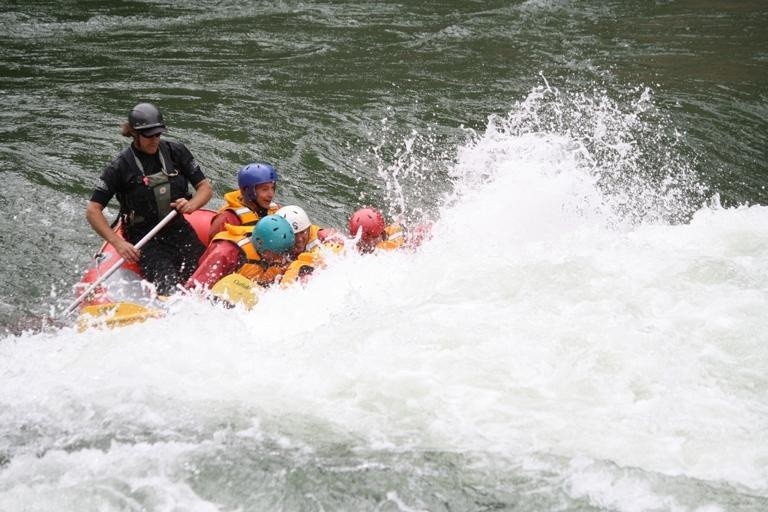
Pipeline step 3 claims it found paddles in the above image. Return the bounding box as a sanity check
[78,274,269,329]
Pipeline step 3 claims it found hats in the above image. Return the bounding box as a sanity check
[142,126,168,136]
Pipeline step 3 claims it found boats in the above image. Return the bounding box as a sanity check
[72,207,435,339]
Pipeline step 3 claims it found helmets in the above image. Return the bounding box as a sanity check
[238,163,277,201]
[130,103,165,129]
[252,215,296,255]
[350,208,384,239]
[274,206,310,234]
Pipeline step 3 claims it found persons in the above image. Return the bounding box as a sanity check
[86,103,214,295]
[184,162,436,310]
